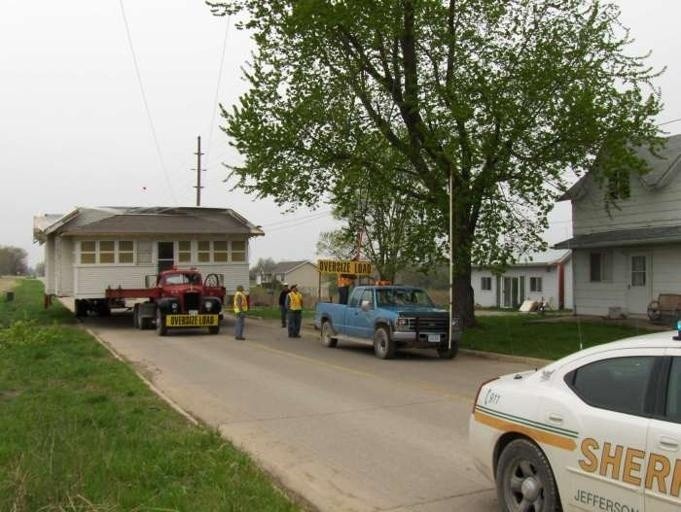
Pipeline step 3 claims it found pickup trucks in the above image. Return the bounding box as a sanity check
[315,285,458,360]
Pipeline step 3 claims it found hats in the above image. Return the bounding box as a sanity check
[290,284,297,289]
[283,283,288,287]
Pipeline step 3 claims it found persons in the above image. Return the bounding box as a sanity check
[284,285,304,338]
[232,284,248,341]
[279,285,291,328]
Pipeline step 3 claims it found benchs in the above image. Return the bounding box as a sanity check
[646,293,681,325]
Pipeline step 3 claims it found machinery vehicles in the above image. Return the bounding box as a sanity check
[75,266,226,336]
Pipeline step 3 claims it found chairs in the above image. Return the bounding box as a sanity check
[599,305,627,327]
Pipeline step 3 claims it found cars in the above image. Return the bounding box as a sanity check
[467,331,681,512]
